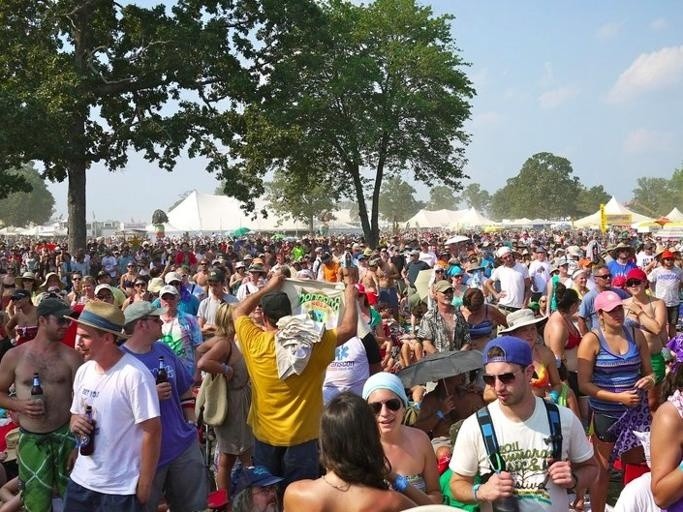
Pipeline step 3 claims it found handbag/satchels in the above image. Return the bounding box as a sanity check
[194,372,228,427]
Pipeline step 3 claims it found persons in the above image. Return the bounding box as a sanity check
[2,227,683,511]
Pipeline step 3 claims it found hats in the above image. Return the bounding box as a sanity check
[482,336,539,380]
[2,427,20,463]
[499,308,547,334]
[232,465,285,494]
[407,243,683,313]
[0,254,386,339]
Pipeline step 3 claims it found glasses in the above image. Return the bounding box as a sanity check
[367,399,401,414]
[482,369,523,384]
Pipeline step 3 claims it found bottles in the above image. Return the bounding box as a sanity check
[79,406,95,456]
[246,285,250,296]
[156,355,169,385]
[30,372,44,416]
